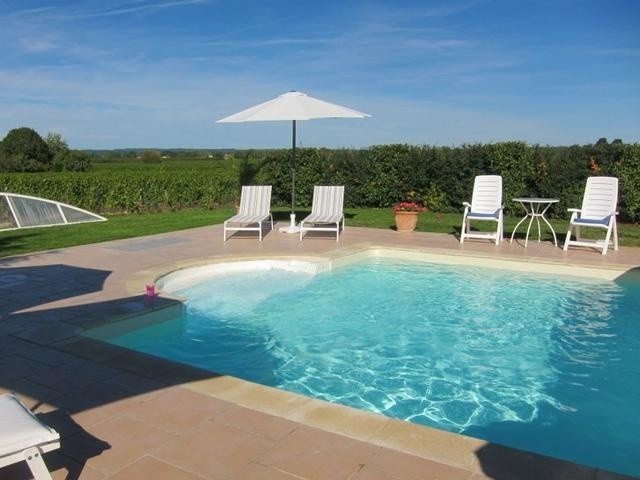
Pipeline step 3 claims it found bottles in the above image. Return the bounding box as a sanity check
[147,281,156,306]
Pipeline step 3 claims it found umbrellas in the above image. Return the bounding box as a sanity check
[215,88,372,214]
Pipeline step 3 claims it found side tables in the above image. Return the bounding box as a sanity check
[509,198,560,247]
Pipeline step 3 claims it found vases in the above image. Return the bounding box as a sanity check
[395,211,418,232]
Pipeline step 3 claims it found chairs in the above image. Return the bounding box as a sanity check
[563,176,620,255]
[459,174,505,246]
[0,391,61,479]
[223,185,272,242]
[299,185,345,244]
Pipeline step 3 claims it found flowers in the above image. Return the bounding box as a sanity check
[393,202,428,213]
